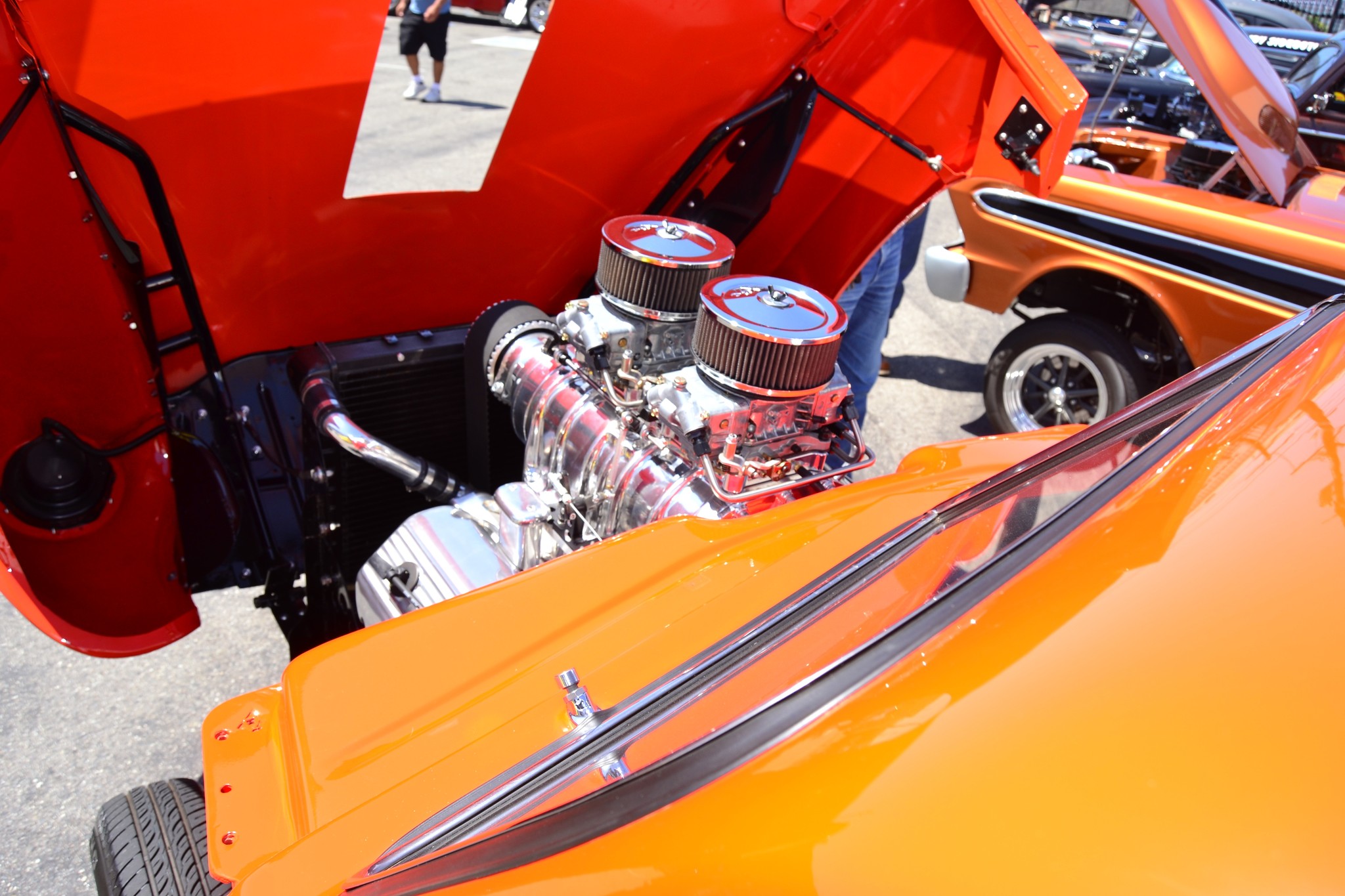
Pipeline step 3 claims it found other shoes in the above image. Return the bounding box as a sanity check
[402,80,426,99]
[422,89,441,102]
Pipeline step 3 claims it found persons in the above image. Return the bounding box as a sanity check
[878,198,930,375]
[395,0,453,103]
[825,223,905,471]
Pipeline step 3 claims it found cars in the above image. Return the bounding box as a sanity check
[923,0,1345,432]
[0,0,1345,894]
[387,0,555,34]
[1024,0,1345,201]
